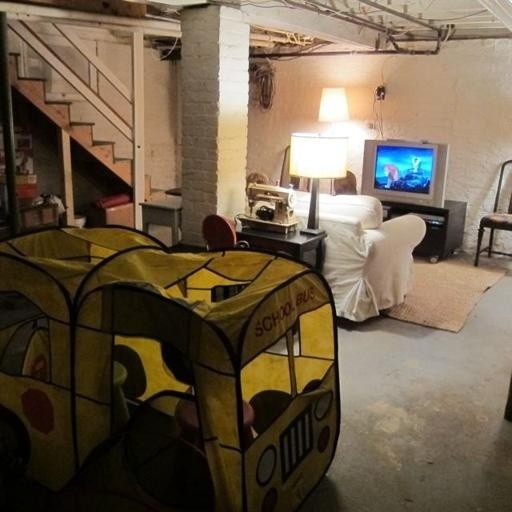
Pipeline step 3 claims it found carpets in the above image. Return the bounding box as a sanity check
[381,259,508,333]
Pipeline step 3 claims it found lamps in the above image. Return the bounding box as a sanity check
[290,133,349,236]
[317,87,350,124]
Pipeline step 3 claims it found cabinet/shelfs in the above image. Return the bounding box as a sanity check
[86,202,135,229]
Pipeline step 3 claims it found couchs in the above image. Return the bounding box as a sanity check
[293,212,426,324]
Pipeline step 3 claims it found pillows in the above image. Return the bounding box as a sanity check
[288,189,386,230]
[95,191,132,210]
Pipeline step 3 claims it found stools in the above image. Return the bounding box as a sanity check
[112,361,133,424]
[175,386,255,479]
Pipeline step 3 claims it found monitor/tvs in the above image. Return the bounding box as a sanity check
[360,139,451,209]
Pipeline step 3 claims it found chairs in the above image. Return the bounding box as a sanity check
[202,215,249,299]
[473,160,512,266]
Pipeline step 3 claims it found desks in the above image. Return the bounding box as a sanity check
[381,200,466,263]
[139,193,183,248]
[235,226,327,336]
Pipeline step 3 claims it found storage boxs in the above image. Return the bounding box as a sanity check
[16,176,38,200]
